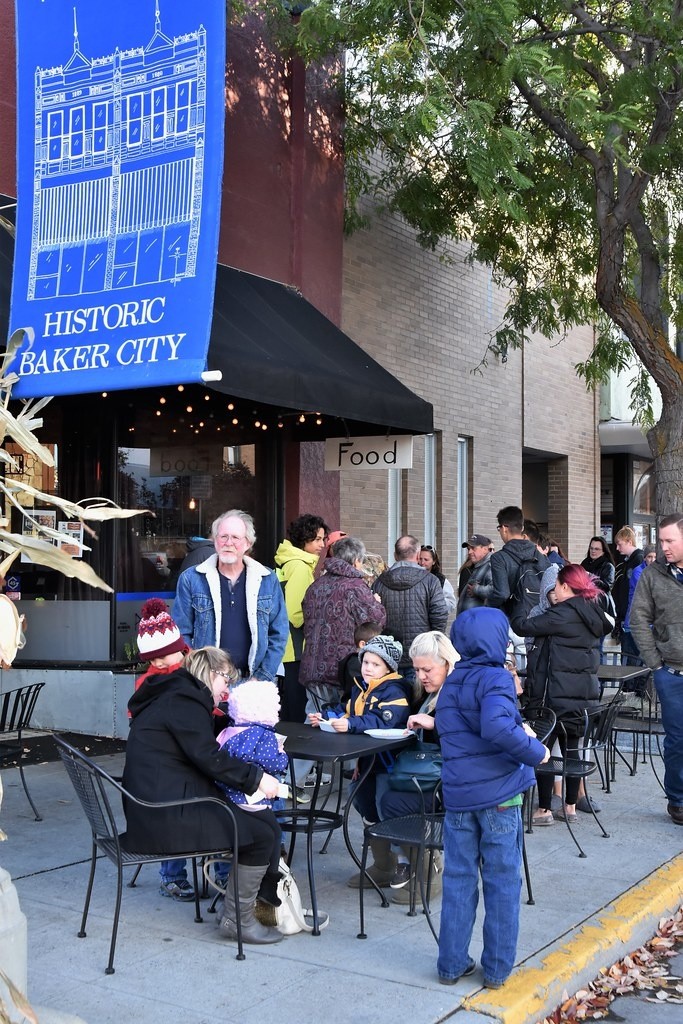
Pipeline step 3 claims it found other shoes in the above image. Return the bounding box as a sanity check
[484,978,501,989]
[667,803,683,824]
[550,794,562,811]
[576,795,601,812]
[524,813,554,825]
[344,770,355,779]
[557,808,578,823]
[438,956,477,985]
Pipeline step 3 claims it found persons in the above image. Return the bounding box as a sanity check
[270,514,332,803]
[436,607,551,988]
[348,631,460,904]
[301,530,455,779]
[308,635,415,889]
[629,513,683,825]
[128,598,233,903]
[458,506,656,823]
[171,510,288,861]
[118,646,284,943]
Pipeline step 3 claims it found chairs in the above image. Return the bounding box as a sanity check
[51,647,666,975]
[1,683,45,822]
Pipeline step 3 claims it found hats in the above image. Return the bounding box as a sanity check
[358,635,403,672]
[137,598,184,661]
[643,543,657,557]
[462,535,488,547]
[326,531,349,547]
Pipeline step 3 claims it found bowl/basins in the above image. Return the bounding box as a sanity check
[317,720,338,733]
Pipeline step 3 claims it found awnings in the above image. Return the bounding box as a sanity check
[1,196,434,445]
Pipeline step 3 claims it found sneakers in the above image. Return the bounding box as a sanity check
[389,862,415,888]
[304,766,332,787]
[284,782,311,803]
[159,877,198,901]
[216,879,228,891]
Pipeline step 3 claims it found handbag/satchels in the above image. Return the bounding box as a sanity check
[388,743,442,792]
[277,856,329,935]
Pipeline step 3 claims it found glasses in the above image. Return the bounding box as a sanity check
[488,548,495,552]
[216,534,246,543]
[590,546,603,550]
[497,525,508,531]
[210,668,232,687]
[421,545,435,555]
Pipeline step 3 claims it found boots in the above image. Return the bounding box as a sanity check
[391,843,441,904]
[257,869,283,907]
[216,860,283,944]
[346,816,398,888]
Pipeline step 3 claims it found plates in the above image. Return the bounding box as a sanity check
[364,728,416,739]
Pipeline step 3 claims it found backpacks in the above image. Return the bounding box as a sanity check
[502,545,541,617]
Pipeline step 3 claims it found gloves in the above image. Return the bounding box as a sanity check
[466,582,477,597]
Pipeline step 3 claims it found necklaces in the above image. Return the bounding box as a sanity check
[215,681,288,925]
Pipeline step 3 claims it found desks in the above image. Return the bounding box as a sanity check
[517,665,652,790]
[274,723,416,936]
